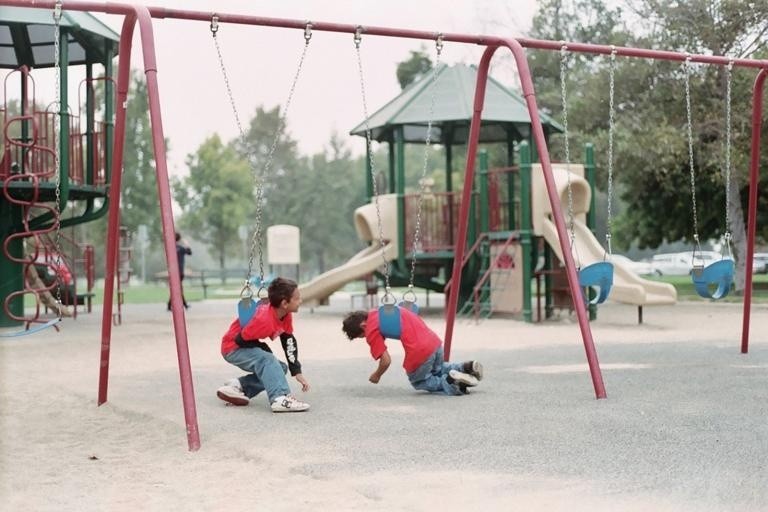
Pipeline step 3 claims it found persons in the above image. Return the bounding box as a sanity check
[341,303,485,396]
[167,232,193,311]
[215,275,311,413]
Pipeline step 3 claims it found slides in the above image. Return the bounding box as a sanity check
[531,218,678,305]
[298,242,400,309]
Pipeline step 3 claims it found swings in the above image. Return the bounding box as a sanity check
[353,34,443,340]
[210,22,312,330]
[685,57,734,300]
[0,2,63,338]
[559,45,615,305]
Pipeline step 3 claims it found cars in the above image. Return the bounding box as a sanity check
[635,251,768,276]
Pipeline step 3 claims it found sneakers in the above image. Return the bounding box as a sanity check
[448,370,478,387]
[465,361,482,381]
[271,394,310,412]
[218,385,249,405]
